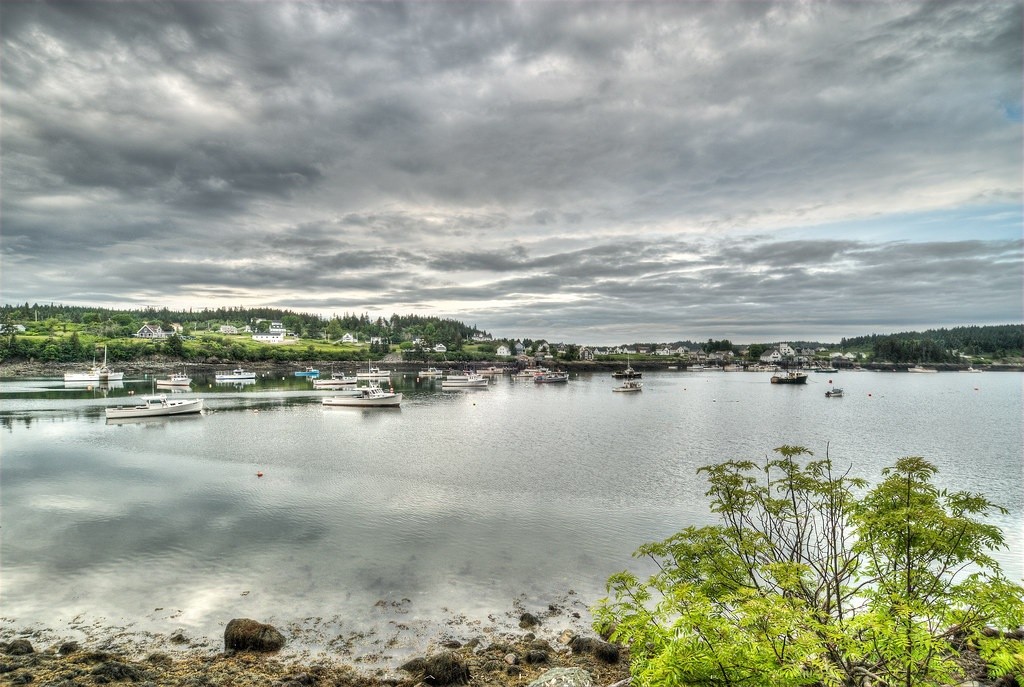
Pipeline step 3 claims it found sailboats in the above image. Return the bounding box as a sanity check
[64,382,124,397]
[611,353,642,379]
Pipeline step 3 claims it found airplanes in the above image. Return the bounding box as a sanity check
[64,345,124,381]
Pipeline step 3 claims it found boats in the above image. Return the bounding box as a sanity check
[825,388,844,397]
[959,367,983,373]
[156,384,192,393]
[770,372,808,384]
[801,362,838,373]
[156,373,192,386]
[313,384,357,391]
[613,380,644,392]
[686,362,782,372]
[215,368,256,379]
[294,367,320,376]
[356,377,391,383]
[908,366,938,373]
[312,359,403,406]
[105,394,204,418]
[215,379,255,390]
[418,366,569,388]
[103,412,200,425]
